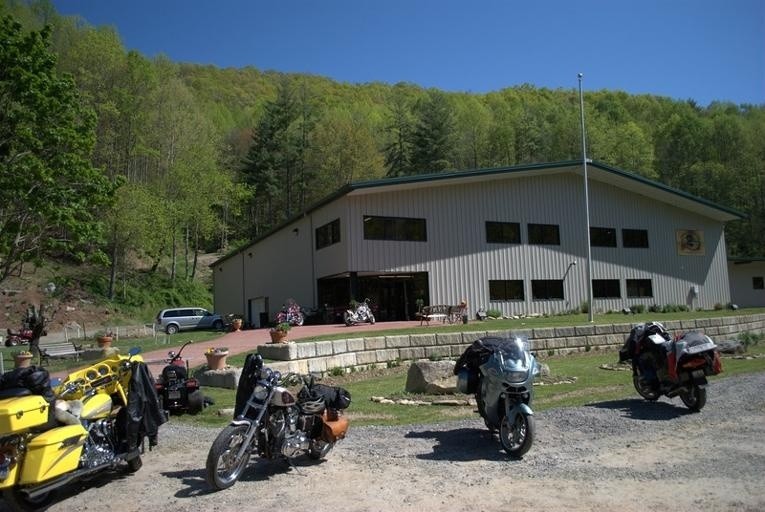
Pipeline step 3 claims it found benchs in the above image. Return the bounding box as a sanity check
[414,303,468,326]
[37,342,85,368]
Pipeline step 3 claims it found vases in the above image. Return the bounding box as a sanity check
[233,319,242,332]
[97,337,113,348]
[15,356,33,368]
[206,352,228,370]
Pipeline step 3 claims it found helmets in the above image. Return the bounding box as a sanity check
[298,396,325,414]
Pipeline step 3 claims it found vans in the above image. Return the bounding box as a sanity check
[155,307,225,335]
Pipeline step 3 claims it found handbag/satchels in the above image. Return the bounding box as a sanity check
[1,365,50,392]
[301,384,351,409]
[321,416,348,442]
[163,366,185,378]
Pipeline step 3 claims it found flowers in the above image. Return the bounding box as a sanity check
[15,350,32,355]
[206,345,228,353]
[97,328,114,337]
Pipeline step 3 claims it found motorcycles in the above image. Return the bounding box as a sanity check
[342,297,376,327]
[274,298,305,326]
[154,339,217,416]
[203,352,352,492]
[451,335,537,460]
[617,319,723,413]
[0,346,171,512]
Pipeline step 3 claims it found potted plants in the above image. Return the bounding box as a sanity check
[269,321,292,343]
